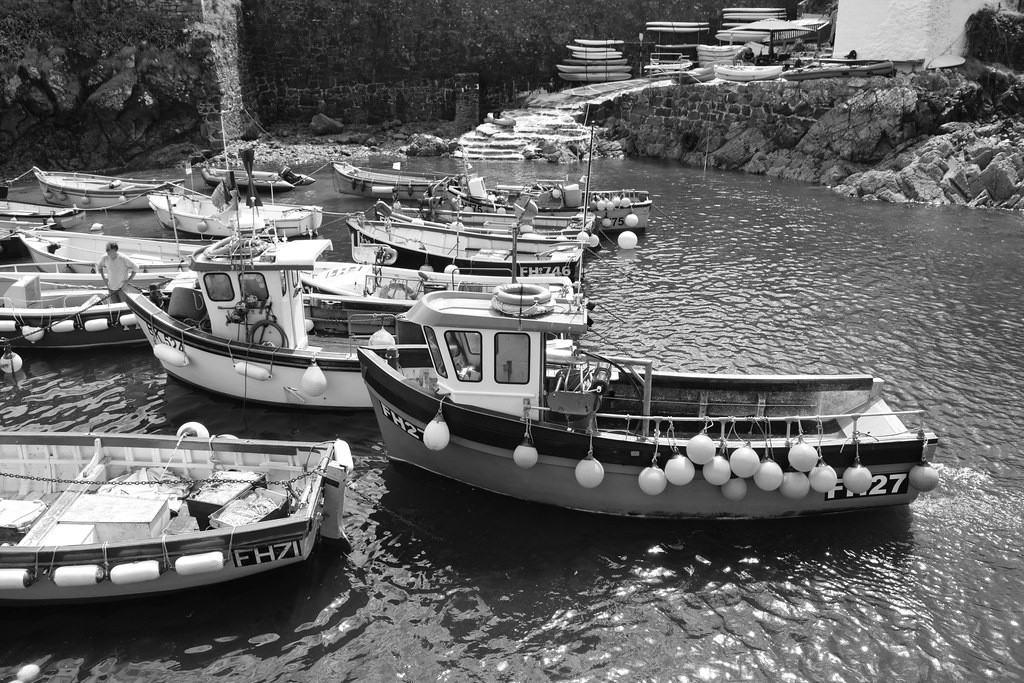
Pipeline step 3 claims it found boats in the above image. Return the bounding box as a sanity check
[0,149,949,609]
[645,47,896,87]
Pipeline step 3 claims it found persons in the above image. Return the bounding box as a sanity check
[97,242,138,302]
[742,47,754,63]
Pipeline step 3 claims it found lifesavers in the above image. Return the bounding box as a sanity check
[494,283,551,304]
[490,297,556,316]
[380,282,418,300]
[234,239,264,253]
[210,235,239,257]
